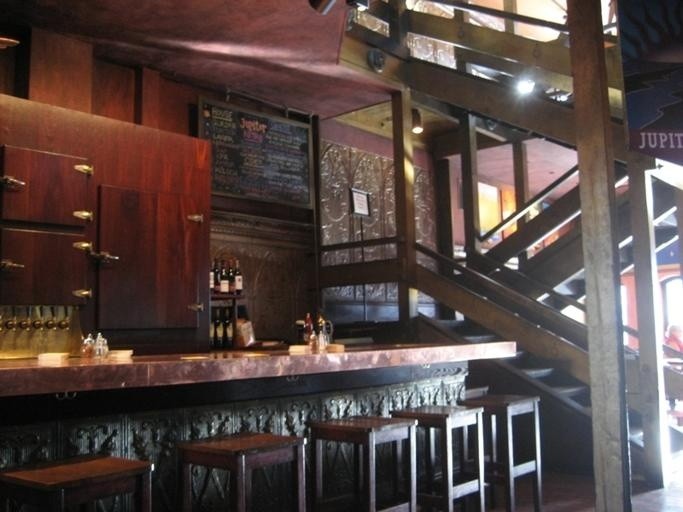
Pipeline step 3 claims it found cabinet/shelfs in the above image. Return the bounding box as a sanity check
[1,91,212,353]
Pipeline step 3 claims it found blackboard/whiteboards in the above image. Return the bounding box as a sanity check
[198,96,315,211]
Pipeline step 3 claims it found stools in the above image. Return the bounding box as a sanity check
[173,430,308,511]
[304,415,420,511]
[389,403,487,512]
[1,453,155,512]
[458,392,545,512]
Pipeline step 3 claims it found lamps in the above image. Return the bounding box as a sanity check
[410,109,424,134]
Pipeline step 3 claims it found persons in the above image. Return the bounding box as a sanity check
[665,324,683,353]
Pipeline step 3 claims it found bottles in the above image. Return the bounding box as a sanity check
[317,314,330,351]
[79,333,94,359]
[209,259,244,296]
[303,311,313,345]
[210,307,234,348]
[94,332,108,360]
